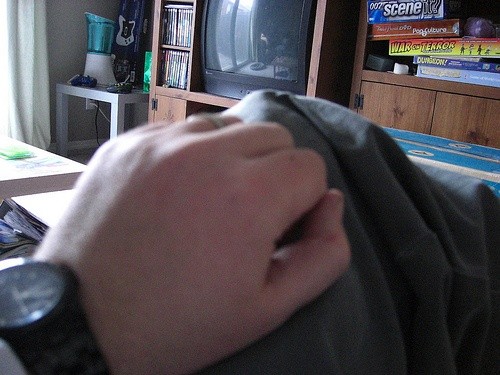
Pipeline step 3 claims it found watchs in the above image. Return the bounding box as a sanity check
[1,257,113,374]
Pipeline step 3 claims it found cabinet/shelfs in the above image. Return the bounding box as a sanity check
[349,0,500,149]
[149,0,359,125]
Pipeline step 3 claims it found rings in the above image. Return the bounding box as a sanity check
[202,112,225,129]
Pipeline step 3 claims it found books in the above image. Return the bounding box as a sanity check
[159,50,190,90]
[160,8,193,48]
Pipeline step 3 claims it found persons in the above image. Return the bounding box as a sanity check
[0,88,500,375]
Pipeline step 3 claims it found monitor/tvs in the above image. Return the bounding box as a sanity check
[200,0,317,101]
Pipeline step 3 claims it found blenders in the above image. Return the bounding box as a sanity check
[83,12,117,88]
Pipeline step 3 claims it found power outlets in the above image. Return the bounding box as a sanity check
[86,98,95,110]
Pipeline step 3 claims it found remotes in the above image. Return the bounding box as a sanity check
[107,83,133,93]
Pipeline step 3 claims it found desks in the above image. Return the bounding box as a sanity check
[55,83,150,157]
